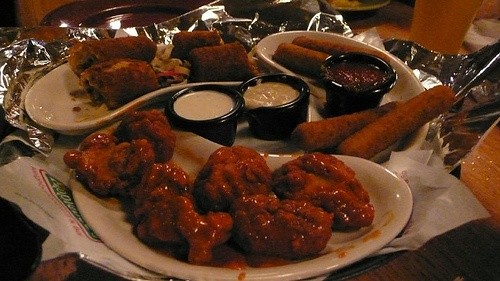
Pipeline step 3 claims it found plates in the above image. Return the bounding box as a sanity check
[39,0,215,28]
[26,62,261,133]
[330,0,390,16]
[69,121,413,281]
[254,30,429,166]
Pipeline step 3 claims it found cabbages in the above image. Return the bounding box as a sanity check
[148,43,191,75]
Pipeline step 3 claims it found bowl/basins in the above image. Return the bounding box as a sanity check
[163,84,245,147]
[320,51,398,114]
[239,73,310,141]
